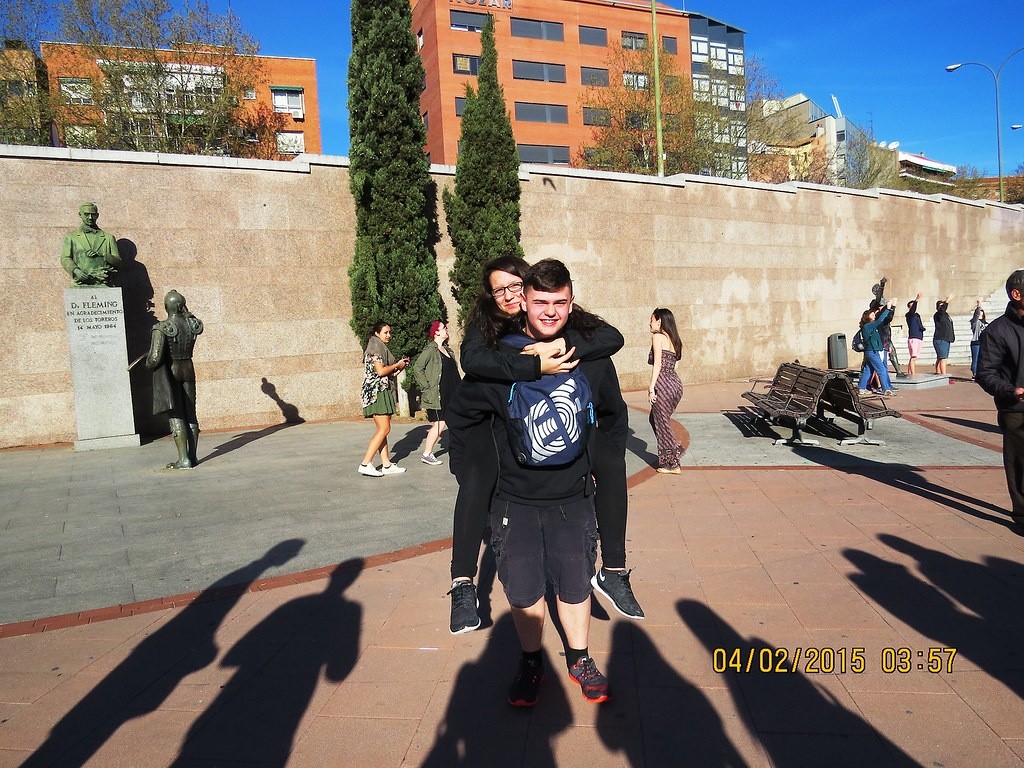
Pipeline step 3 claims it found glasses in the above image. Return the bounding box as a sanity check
[491,282,525,298]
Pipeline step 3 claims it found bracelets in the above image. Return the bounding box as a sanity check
[649,392,653,394]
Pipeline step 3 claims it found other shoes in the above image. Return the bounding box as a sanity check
[657,465,681,475]
[874,387,884,394]
[859,387,872,395]
[972,376,976,382]
[892,386,900,392]
[884,391,897,397]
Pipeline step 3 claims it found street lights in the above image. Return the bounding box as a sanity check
[945,48,1024,203]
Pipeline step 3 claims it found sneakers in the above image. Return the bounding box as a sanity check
[591,567,645,620]
[382,462,407,474]
[358,463,384,477]
[568,655,614,703]
[447,577,481,635]
[421,452,443,465]
[507,659,546,707]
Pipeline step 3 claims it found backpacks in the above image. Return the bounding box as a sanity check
[852,324,874,352]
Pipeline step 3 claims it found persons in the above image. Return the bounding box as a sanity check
[444,259,629,707]
[451,259,647,635]
[905,294,923,375]
[975,270,1024,534]
[871,276,907,377]
[414,321,461,465]
[648,307,685,475]
[969,300,989,381]
[858,298,901,396]
[145,290,203,468]
[357,321,410,477]
[60,202,122,285]
[933,294,955,375]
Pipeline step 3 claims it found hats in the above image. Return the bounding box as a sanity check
[430,321,440,338]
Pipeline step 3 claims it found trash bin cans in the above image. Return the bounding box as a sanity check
[829,333,848,369]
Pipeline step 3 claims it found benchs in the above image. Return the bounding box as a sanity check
[740,361,901,447]
